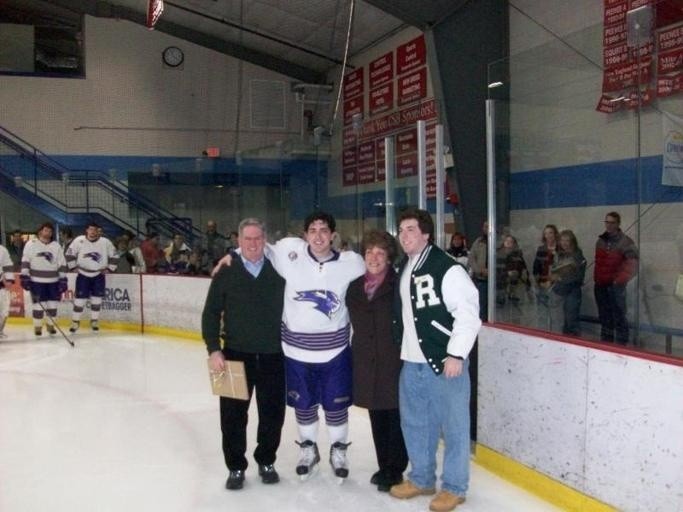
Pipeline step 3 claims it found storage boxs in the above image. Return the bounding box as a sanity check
[578,312,683,357]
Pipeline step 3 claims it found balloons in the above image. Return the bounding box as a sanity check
[162,45,183,67]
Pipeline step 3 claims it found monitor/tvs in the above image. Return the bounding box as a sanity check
[206,358,250,402]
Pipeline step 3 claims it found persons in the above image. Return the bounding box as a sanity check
[64,222,123,334]
[200,216,289,491]
[0,243,18,340]
[344,229,409,493]
[18,220,70,337]
[208,210,370,482]
[443,210,641,348]
[387,207,482,512]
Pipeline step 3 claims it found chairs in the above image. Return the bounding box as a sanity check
[295,440,320,474]
[430,492,465,511]
[227,470,245,489]
[391,481,437,497]
[258,465,280,483]
[329,441,352,477]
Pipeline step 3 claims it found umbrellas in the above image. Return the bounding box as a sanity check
[379,474,403,492]
